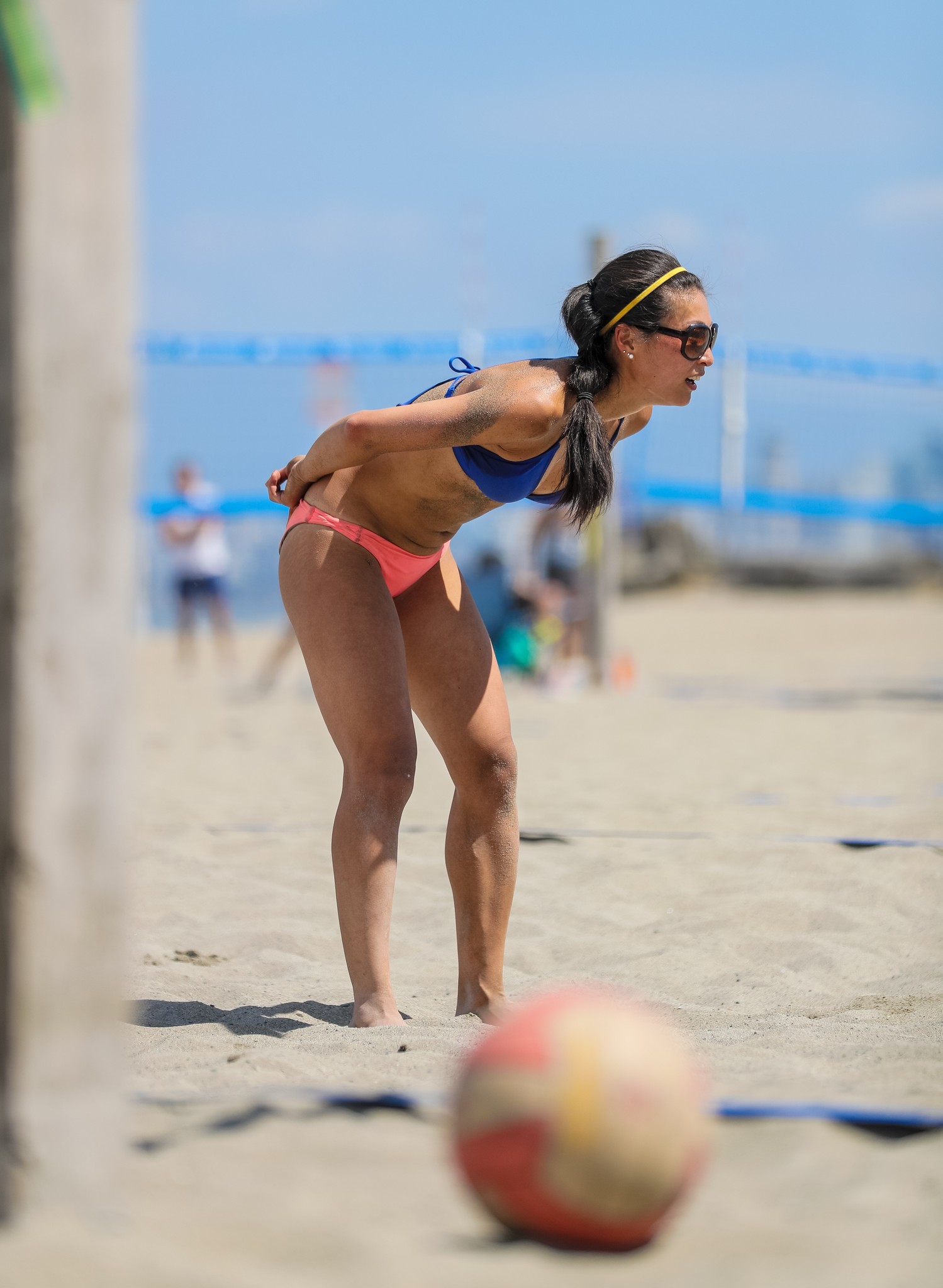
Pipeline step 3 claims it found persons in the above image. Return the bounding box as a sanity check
[268,247,713,1025]
[160,457,234,663]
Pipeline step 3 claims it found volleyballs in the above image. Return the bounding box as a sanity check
[451,988,716,1249]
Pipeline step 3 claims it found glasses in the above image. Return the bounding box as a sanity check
[627,318,718,361]
